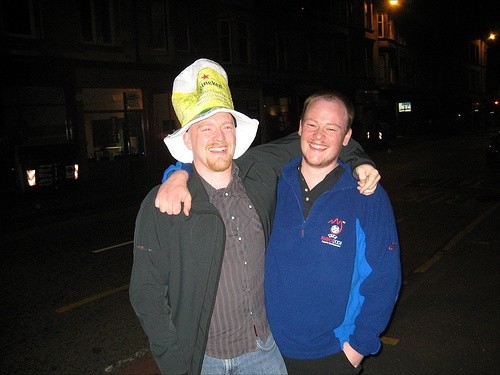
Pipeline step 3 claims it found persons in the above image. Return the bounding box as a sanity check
[154,91,402,375]
[128,58,382,375]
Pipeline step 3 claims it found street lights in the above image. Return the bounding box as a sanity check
[480,30,495,94]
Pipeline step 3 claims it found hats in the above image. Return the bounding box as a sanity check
[163,58,259,163]
